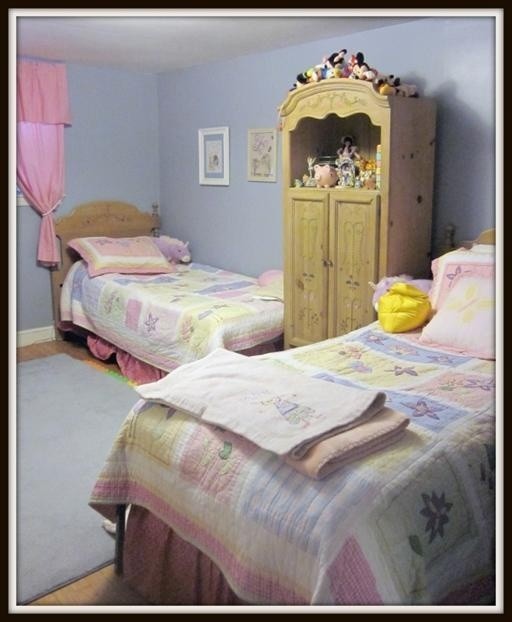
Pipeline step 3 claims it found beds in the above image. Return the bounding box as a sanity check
[49,201,284,384]
[113,228,496,604]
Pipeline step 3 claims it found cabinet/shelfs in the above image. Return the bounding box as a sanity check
[276,78,435,350]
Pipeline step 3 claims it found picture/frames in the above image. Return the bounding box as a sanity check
[247,127,278,183]
[198,125,229,186]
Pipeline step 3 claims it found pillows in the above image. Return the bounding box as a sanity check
[427,247,497,320]
[417,272,496,360]
[67,236,178,277]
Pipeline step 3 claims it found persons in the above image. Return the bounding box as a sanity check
[335,136,361,188]
[302,155,316,188]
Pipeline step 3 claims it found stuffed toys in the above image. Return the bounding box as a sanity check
[290,50,419,98]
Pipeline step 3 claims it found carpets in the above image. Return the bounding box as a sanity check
[14,353,142,606]
